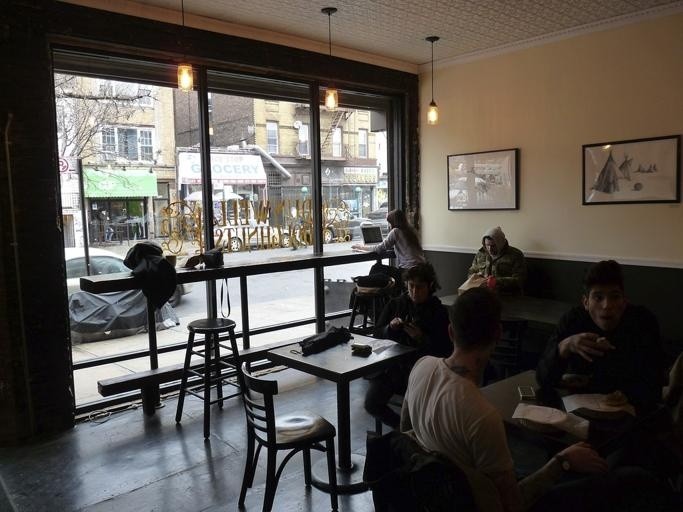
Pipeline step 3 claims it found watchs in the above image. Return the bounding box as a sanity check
[552,454,571,480]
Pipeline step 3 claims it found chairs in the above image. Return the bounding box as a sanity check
[230,359,339,512]
[358,434,469,511]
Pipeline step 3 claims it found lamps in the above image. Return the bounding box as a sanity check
[424,35,440,124]
[172,0,196,93]
[318,5,341,111]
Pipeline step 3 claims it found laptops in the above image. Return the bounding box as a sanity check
[360,226,384,248]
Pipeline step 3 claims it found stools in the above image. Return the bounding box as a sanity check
[173,317,246,440]
[347,290,386,336]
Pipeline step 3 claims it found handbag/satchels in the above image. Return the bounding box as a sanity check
[357,276,392,293]
[201,250,225,265]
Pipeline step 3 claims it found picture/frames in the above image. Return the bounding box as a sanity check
[581,133,679,207]
[445,148,519,210]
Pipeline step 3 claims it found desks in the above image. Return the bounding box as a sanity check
[74,247,394,409]
[478,364,680,463]
[264,329,418,496]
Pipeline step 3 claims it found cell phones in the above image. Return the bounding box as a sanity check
[517,384,537,401]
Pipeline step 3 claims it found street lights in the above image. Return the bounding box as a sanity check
[299,186,307,201]
[353,186,361,217]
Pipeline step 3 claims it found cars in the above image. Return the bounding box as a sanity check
[380,201,388,210]
[198,218,298,253]
[63,246,192,311]
[364,206,389,236]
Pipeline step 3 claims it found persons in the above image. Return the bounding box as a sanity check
[467,225,531,296]
[365,263,452,432]
[99,211,114,242]
[351,208,429,270]
[400,287,605,512]
[535,259,683,486]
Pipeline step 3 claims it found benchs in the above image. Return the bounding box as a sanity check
[440,288,578,335]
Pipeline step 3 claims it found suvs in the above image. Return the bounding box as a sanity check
[288,206,375,245]
